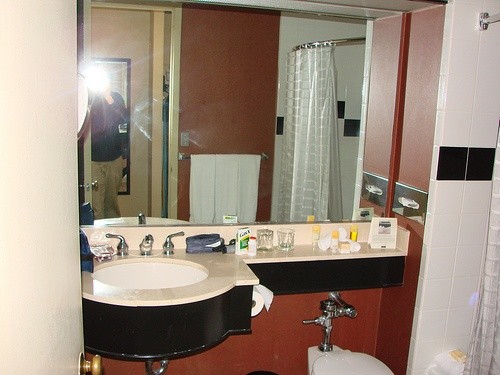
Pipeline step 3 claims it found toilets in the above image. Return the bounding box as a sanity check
[307,343,395,375]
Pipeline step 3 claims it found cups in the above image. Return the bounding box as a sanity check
[257,229,273,252]
[277,228,295,250]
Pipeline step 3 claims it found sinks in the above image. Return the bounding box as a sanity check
[81,258,259,362]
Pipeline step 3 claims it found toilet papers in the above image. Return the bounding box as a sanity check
[251,284,274,317]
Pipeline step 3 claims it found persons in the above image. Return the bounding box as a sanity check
[88,65,129,222]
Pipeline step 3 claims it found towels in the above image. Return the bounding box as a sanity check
[317,227,361,254]
[187,154,262,224]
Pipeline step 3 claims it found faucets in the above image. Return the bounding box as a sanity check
[138,212,146,225]
[139,234,154,256]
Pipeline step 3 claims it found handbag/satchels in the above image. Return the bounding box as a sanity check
[186,234,227,254]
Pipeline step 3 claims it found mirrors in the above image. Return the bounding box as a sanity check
[76,0,403,228]
[89,58,131,195]
